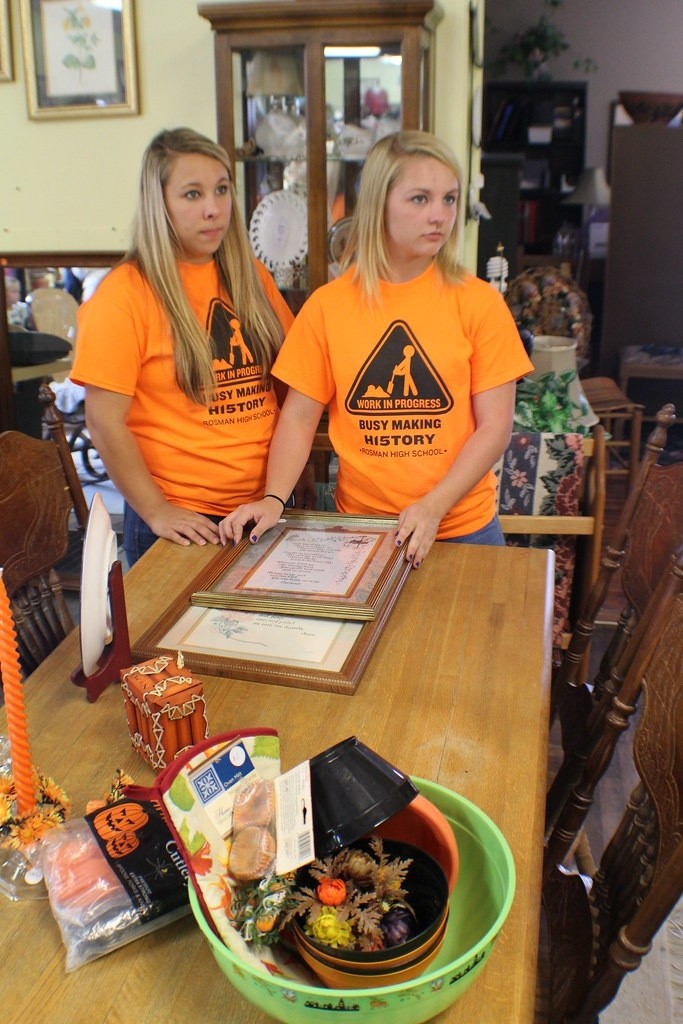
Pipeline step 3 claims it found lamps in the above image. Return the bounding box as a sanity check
[564,167,609,284]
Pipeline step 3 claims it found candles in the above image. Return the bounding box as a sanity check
[0,572,37,814]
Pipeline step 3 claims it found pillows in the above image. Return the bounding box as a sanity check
[7,332,72,365]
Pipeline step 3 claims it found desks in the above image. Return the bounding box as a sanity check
[0,534,555,1023]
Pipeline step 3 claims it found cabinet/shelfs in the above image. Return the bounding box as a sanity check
[198,1,445,485]
[485,78,589,268]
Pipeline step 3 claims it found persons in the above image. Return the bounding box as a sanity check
[363,77,389,120]
[66,129,297,567]
[217,129,535,570]
[6,265,111,414]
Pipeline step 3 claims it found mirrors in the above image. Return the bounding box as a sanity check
[1,250,130,340]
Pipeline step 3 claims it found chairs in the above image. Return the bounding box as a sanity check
[1,380,89,695]
[0,425,683,1024]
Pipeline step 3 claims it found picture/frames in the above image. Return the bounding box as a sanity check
[20,0,139,122]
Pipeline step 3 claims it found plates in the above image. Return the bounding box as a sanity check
[249,189,312,272]
[327,216,359,272]
[79,489,119,679]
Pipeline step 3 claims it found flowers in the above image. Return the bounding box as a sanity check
[493,0,598,72]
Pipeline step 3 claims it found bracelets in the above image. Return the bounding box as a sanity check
[262,494,285,509]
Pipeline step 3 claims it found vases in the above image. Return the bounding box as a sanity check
[516,334,599,428]
[526,63,548,81]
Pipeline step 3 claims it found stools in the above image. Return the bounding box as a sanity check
[614,345,683,458]
[582,377,644,498]
[40,378,109,478]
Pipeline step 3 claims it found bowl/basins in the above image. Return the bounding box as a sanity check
[278,738,461,989]
[187,767,517,1024]
